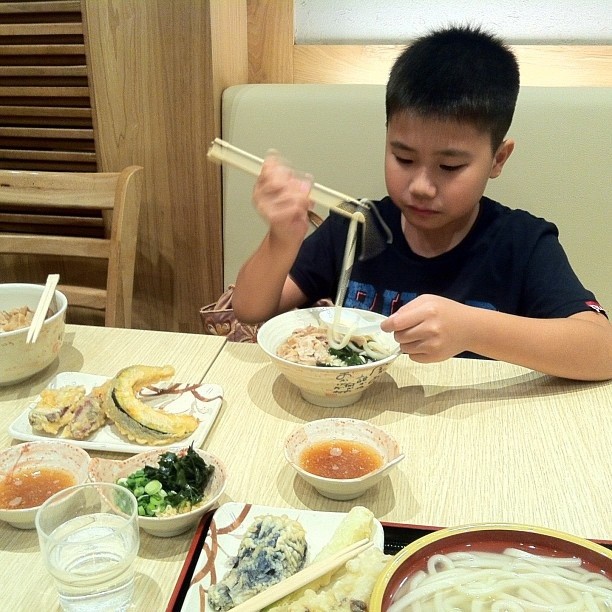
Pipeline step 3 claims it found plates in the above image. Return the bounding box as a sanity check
[180,502,384,612]
[9,371,224,454]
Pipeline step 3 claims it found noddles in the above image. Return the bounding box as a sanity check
[388,544,611,611]
[279,214,397,364]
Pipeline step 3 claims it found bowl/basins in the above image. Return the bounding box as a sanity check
[369,524,612,612]
[88,449,228,537]
[0,283,68,387]
[257,306,402,408]
[1,441,90,529]
[284,417,405,501]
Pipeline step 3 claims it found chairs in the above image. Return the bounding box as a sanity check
[1,165,144,329]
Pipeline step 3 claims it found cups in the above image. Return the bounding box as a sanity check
[35,482,140,612]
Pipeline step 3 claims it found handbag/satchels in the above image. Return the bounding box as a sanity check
[200,210,335,344]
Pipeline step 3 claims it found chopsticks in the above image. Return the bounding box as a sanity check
[227,537,377,612]
[206,138,370,223]
[26,274,60,343]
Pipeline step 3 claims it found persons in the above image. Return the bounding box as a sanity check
[231,23,611,382]
